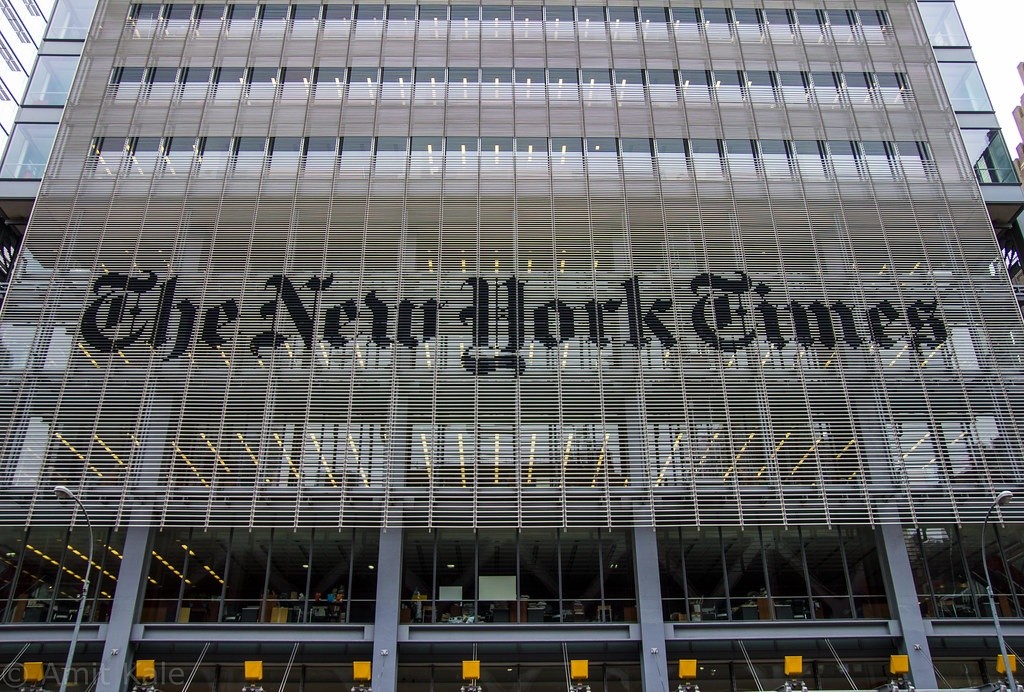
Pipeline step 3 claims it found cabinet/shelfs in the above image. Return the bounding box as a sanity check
[13,589,1012,623]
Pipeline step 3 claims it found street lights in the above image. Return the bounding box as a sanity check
[53,486,93,692]
[982,490,1017,692]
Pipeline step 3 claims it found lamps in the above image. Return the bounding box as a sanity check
[17,655,1016,683]
